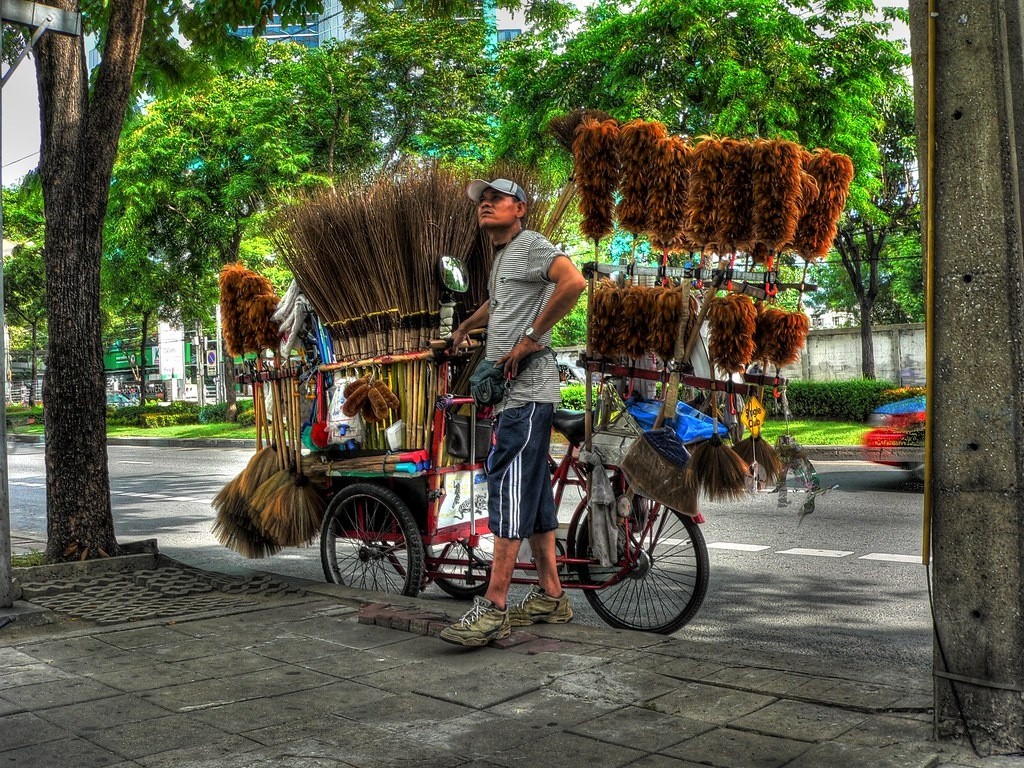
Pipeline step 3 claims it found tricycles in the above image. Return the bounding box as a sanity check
[314,394,709,634]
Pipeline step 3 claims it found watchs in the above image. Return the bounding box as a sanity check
[526,327,541,342]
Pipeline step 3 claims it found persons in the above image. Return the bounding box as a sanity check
[440,177,587,646]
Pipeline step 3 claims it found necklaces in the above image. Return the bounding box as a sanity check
[487,238,511,314]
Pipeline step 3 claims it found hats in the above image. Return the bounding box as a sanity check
[466,178,527,209]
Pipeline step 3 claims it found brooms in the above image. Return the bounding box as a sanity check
[620,261,783,516]
[210,108,624,560]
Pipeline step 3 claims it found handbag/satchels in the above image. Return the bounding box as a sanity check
[468,347,553,408]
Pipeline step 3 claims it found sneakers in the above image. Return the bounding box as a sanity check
[439,596,511,647]
[508,585,574,627]
